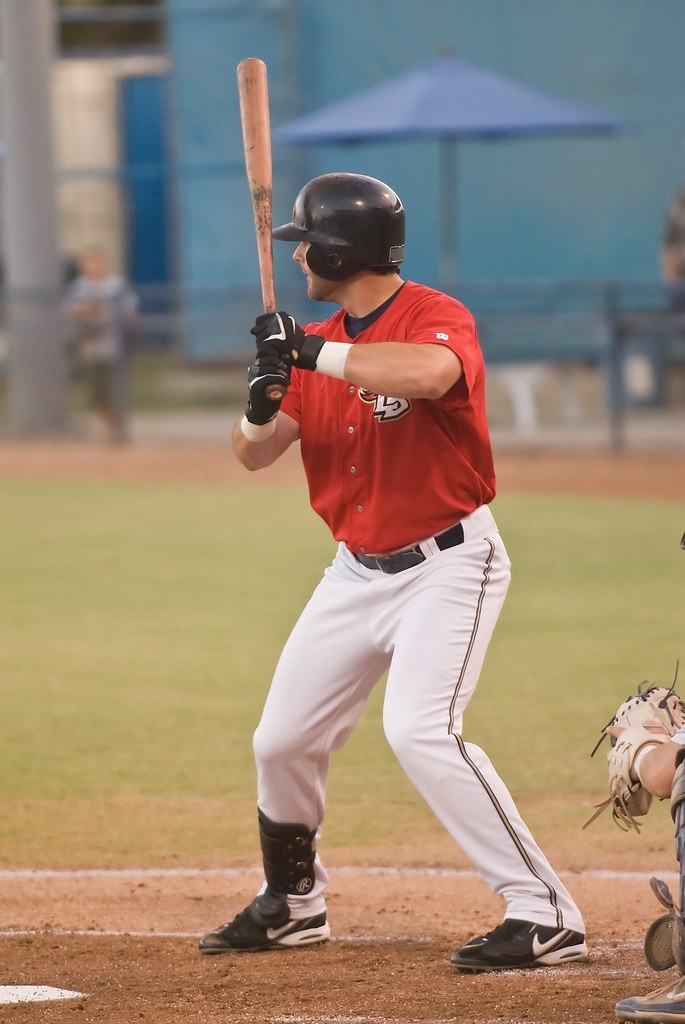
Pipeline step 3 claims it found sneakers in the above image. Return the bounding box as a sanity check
[197,906,330,953]
[450,919,588,969]
[615,974,685,1022]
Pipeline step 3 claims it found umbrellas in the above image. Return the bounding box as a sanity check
[272,57,637,285]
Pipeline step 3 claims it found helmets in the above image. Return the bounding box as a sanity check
[272,173,405,281]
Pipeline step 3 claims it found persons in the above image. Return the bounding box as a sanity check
[198,169,588,976]
[54,235,138,447]
[605,694,685,1024]
[656,186,685,410]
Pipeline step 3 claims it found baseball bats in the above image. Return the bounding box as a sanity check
[237,58,287,400]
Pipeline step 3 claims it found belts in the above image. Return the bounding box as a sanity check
[353,523,464,575]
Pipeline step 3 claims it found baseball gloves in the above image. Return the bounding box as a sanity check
[580,658,685,835]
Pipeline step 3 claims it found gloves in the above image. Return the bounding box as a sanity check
[250,312,325,373]
[244,345,293,425]
